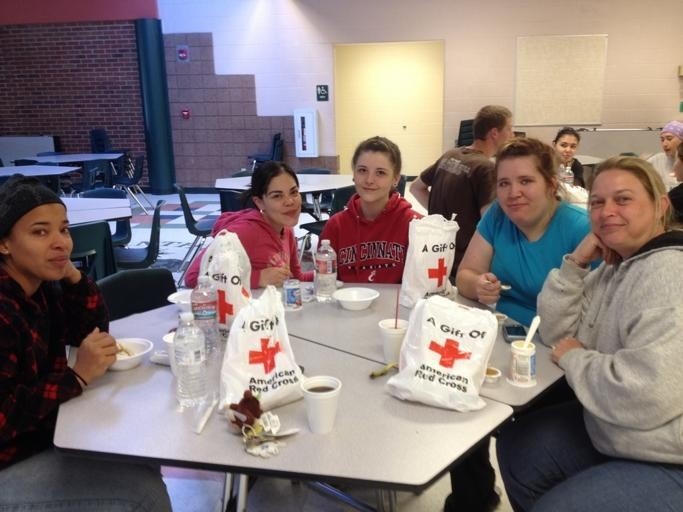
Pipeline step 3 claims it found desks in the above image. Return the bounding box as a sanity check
[569,155,606,177]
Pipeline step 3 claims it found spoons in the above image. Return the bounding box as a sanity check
[486,281,511,290]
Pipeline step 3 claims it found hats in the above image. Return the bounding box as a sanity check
[0,173,67,240]
[660,120,683,142]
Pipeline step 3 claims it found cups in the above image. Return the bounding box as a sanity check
[163,334,176,373]
[511,341,536,383]
[302,376,341,434]
[379,319,405,365]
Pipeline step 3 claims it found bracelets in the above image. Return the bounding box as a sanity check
[70,366,87,385]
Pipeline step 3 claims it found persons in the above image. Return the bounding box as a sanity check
[444,138,616,512]
[496,153,681,510]
[317,134,428,284]
[407,104,516,287]
[551,127,584,189]
[666,142,682,225]
[0,174,174,512]
[183,158,317,290]
[646,120,682,193]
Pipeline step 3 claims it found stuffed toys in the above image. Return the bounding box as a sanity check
[224,388,264,436]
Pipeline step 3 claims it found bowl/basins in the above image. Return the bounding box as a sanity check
[332,288,379,311]
[168,290,192,311]
[108,337,155,372]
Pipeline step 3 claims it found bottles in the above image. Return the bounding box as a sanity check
[172,312,206,404]
[283,278,303,311]
[315,241,338,304]
[190,275,220,366]
[560,165,575,185]
